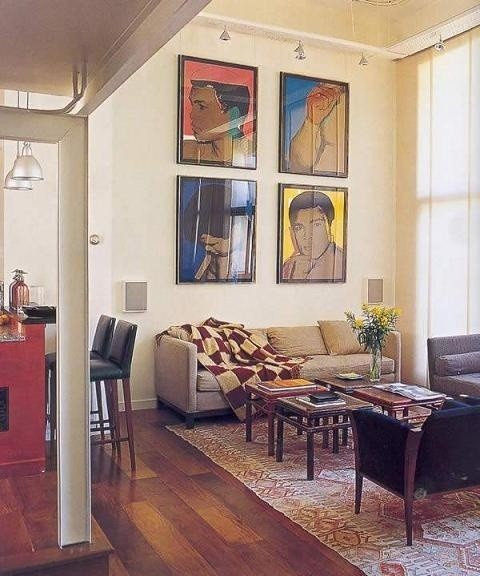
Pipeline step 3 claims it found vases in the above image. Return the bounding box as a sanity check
[368,340,383,383]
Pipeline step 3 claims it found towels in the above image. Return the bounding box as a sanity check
[341,372,360,378]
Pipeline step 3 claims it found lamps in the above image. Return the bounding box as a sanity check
[10,92,44,182]
[4,89,34,191]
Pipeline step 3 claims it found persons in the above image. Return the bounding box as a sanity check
[289,82,347,173]
[183,79,253,165]
[282,190,344,281]
[182,184,243,281]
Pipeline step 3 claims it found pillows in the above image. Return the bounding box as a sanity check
[266,326,326,356]
[318,319,361,357]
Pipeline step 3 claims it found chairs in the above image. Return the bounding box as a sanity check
[44,316,116,452]
[346,399,480,547]
[47,320,137,473]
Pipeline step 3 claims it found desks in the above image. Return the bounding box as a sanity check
[0,324,49,479]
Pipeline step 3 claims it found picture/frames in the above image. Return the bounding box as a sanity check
[279,70,350,179]
[175,175,258,285]
[176,55,259,170]
[276,182,350,284]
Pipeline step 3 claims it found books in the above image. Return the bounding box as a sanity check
[295,395,348,408]
[256,379,317,392]
[371,382,446,401]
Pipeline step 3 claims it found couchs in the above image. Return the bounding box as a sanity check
[427,334,480,398]
[153,325,401,429]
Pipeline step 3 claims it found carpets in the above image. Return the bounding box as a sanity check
[163,421,480,576]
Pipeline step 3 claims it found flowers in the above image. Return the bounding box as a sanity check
[345,304,403,351]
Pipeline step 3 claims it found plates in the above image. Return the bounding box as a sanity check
[334,372,365,380]
[307,390,342,402]
